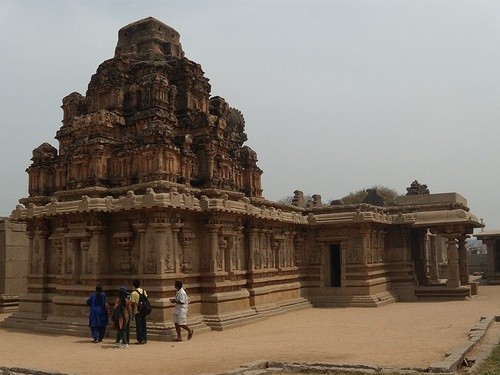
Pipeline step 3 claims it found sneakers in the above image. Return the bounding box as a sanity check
[135,340,148,344]
[172,339,182,341]
[119,343,128,348]
[188,330,193,339]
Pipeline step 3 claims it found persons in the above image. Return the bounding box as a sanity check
[87,285,111,343]
[170,281,193,341]
[114,288,132,347]
[129,280,148,344]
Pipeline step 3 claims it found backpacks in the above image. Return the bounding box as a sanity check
[133,290,152,315]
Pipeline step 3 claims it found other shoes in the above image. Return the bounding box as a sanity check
[94,340,98,343]
[98,339,102,342]
